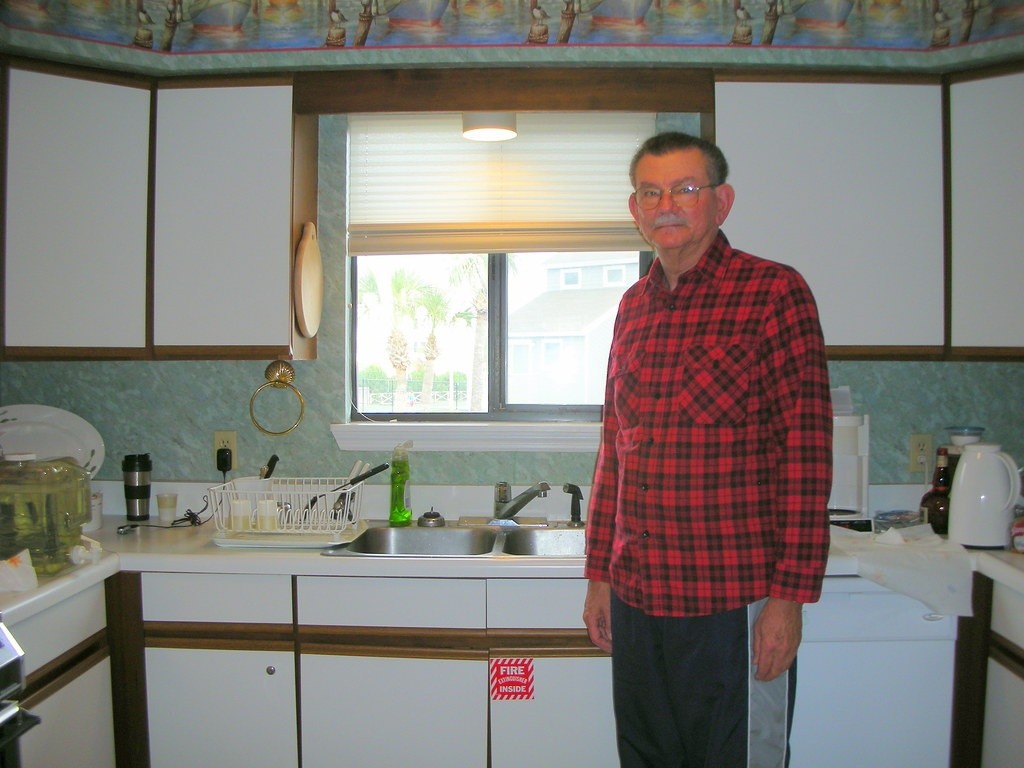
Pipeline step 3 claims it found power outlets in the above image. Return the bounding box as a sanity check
[910,433,933,473]
[213,430,237,470]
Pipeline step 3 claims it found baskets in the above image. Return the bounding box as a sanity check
[210,476,364,534]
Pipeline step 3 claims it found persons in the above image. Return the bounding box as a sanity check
[583,131,835,768]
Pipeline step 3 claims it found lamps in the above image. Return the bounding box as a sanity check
[462,111,518,142]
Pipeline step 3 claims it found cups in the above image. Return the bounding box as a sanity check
[156,492,178,521]
[123,453,152,521]
[256,499,278,531]
[230,499,251,530]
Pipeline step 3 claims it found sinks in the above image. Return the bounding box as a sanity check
[503,529,586,556]
[346,525,495,554]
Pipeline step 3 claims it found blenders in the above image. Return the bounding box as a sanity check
[943,425,985,491]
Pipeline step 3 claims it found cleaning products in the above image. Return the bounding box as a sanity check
[389,439,415,526]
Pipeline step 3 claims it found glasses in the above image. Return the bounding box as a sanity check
[632,184,720,210]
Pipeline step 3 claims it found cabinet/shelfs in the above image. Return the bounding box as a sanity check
[946,54,1024,360]
[151,74,323,362]
[711,72,947,361]
[8,569,622,768]
[965,570,1024,768]
[0,53,151,363]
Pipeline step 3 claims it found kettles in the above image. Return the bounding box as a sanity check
[948,443,1021,551]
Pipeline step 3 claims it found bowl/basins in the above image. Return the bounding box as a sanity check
[873,511,920,531]
[276,501,292,524]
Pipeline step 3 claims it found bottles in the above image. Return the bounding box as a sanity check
[921,447,952,535]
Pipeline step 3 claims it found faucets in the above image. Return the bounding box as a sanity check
[487,481,552,527]
